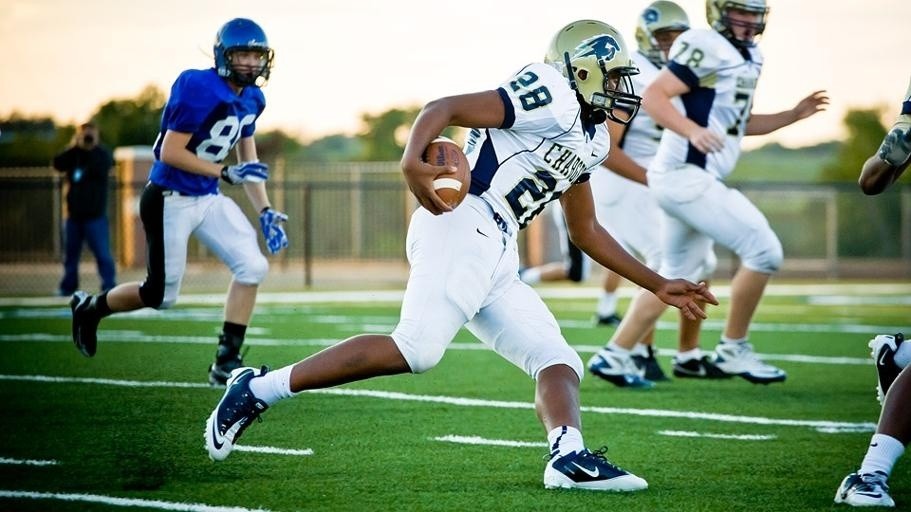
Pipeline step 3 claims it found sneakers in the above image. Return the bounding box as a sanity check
[711,340,788,385]
[205,360,247,390]
[69,289,100,358]
[596,312,622,328]
[203,365,270,463]
[867,333,904,408]
[673,353,732,379]
[542,448,649,494]
[630,344,674,385]
[834,470,897,510]
[587,345,657,389]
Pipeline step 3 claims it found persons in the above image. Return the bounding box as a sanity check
[200,18,718,493]
[68,19,289,390]
[53,122,116,295]
[590,0,733,381]
[516,203,625,326]
[833,76,911,508]
[587,0,830,388]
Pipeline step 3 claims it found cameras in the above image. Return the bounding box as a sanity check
[84,135,93,143]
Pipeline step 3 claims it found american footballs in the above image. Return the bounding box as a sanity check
[422,135,471,210]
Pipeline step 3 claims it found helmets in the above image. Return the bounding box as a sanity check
[705,0,771,49]
[543,18,643,127]
[212,18,276,89]
[634,0,692,69]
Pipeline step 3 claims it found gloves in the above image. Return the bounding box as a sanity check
[257,206,289,255]
[219,158,270,186]
[878,125,911,166]
